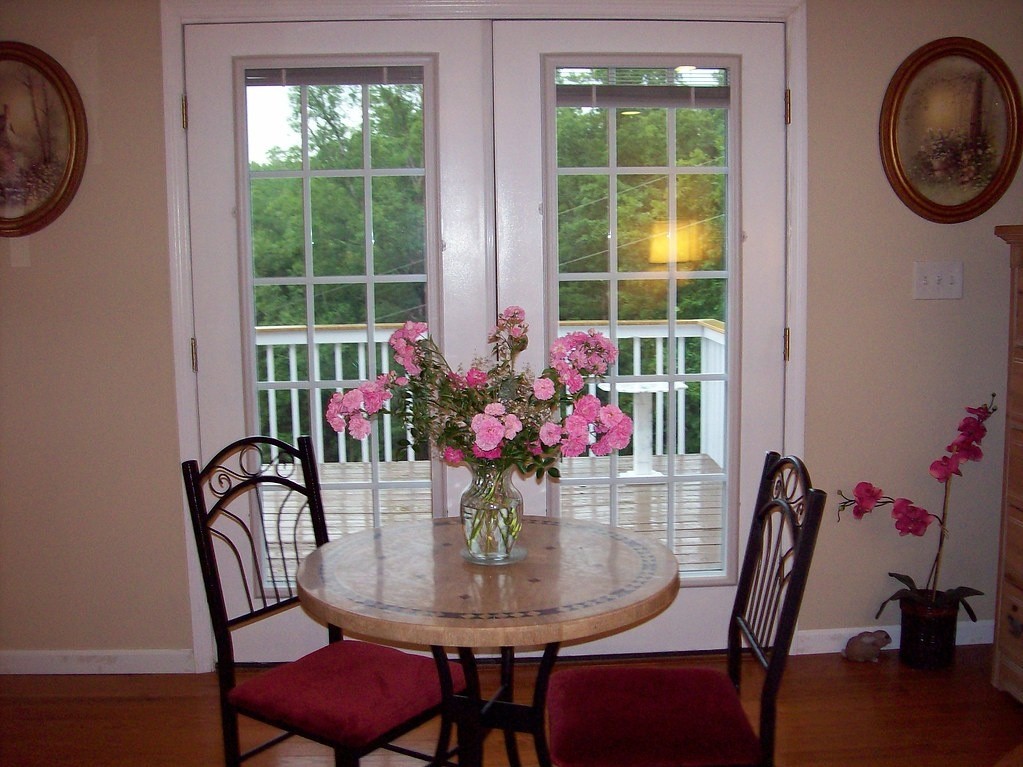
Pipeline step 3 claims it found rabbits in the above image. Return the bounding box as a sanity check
[842,630,893,663]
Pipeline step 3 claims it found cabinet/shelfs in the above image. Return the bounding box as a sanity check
[990,224,1023,703]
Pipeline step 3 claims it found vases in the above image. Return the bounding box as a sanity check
[897,588,959,671]
[458,459,526,565]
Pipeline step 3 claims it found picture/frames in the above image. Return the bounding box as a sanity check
[0,40,89,236]
[879,36,1023,224]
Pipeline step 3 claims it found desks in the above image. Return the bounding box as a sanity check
[296,516,680,767]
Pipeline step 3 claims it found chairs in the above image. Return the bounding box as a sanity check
[547,451,828,766]
[181,434,466,767]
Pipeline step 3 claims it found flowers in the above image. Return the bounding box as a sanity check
[324,305,634,554]
[833,393,1000,623]
